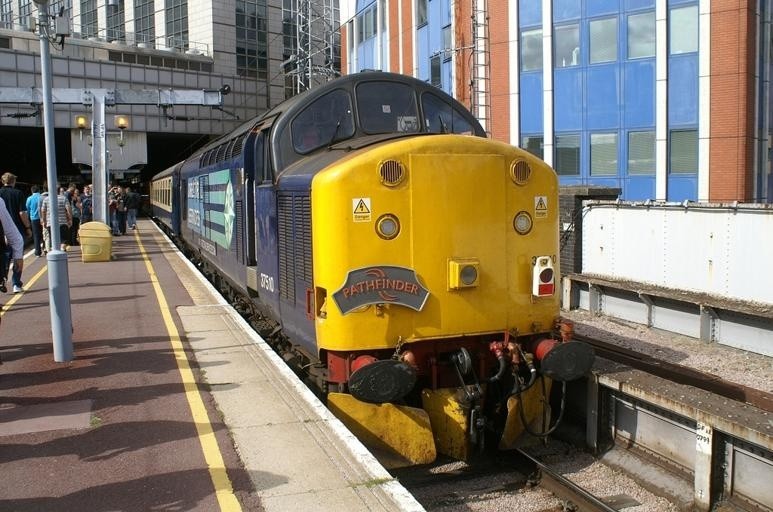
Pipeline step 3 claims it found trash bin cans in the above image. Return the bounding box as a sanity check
[79,221,113,262]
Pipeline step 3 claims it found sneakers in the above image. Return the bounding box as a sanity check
[128,224,136,230]
[13,284,24,293]
[0,277,7,294]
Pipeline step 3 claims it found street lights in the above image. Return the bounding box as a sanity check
[75,113,128,226]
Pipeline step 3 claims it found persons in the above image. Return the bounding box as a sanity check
[0,172,139,293]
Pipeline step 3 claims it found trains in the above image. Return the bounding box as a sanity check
[148,67,596,473]
